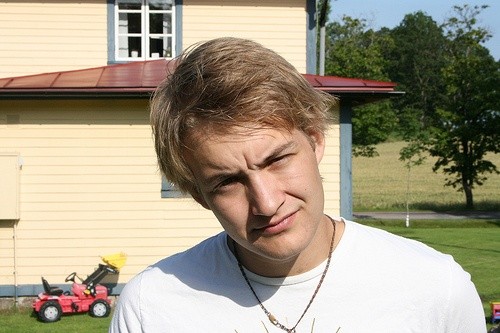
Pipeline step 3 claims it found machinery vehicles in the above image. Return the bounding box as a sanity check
[31,272,111,324]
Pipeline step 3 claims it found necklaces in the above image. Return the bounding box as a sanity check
[235,214,336,333]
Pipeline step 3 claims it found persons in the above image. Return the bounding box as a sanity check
[107,37,487,333]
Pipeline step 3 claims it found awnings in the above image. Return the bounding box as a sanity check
[0,59,405,98]
